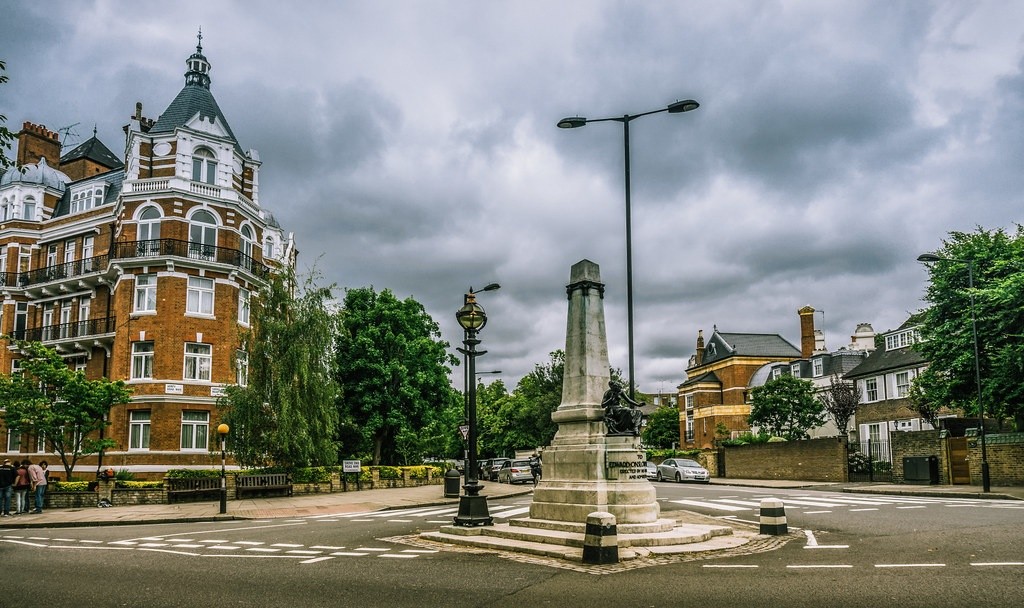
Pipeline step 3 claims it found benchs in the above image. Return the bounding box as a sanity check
[238,473,294,499]
[167,478,221,504]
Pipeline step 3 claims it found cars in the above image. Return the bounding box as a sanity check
[646,461,658,481]
[497,458,536,485]
[656,458,710,485]
[476,459,489,480]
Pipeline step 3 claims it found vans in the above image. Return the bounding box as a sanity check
[483,457,511,482]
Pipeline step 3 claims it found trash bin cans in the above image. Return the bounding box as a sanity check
[444,468,460,498]
[903,455,938,485]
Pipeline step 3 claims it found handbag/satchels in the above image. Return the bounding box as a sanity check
[105,470,115,478]
[88,482,98,491]
[97,498,113,508]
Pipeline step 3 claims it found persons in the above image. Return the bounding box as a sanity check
[0,459,49,517]
[529,454,542,483]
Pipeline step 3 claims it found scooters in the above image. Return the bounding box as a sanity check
[530,464,541,486]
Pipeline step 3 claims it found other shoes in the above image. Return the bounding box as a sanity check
[30,511,42,514]
[22,511,27,514]
[4,513,11,517]
[15,513,21,515]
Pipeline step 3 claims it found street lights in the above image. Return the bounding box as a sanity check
[217,423,230,514]
[557,99,701,407]
[454,283,502,527]
[916,253,991,493]
